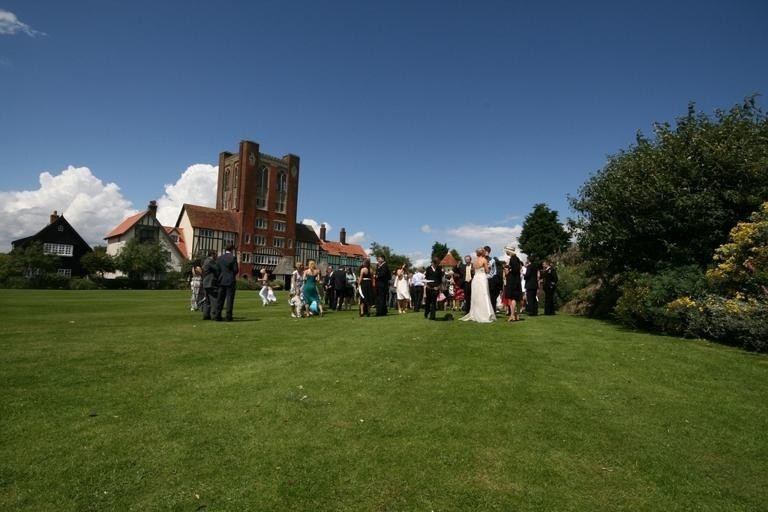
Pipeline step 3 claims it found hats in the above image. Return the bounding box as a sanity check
[503,245,517,255]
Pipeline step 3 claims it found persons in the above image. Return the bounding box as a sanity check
[267,285,277,303]
[190,259,202,311]
[214,245,239,321]
[289,246,558,323]
[258,269,269,306]
[202,252,217,320]
